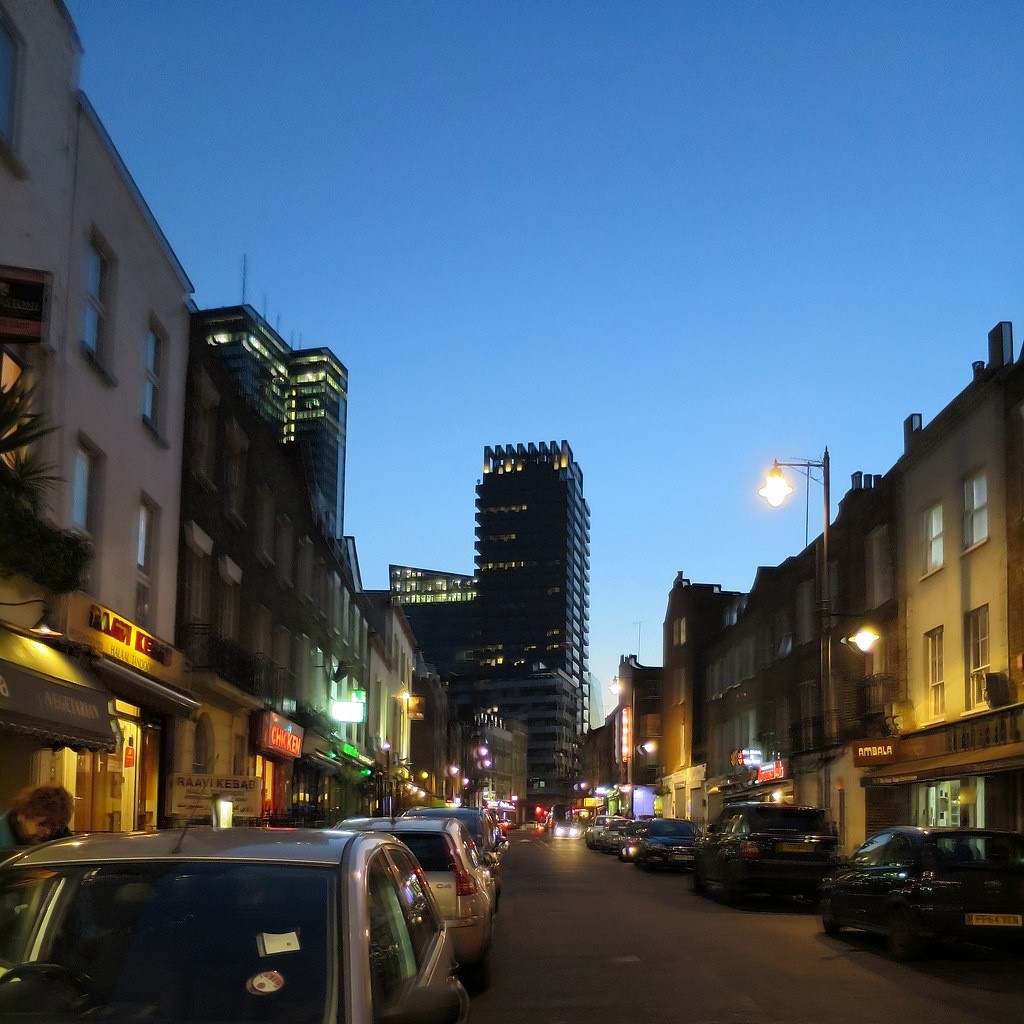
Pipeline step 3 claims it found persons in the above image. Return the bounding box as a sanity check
[0,786,73,852]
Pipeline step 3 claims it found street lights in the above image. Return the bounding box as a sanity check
[609,674,636,814]
[757,447,834,814]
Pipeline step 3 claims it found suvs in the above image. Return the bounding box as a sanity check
[691,801,840,907]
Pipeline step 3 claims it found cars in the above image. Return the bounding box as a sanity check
[555,820,584,839]
[586,815,626,849]
[600,819,631,852]
[525,821,541,830]
[0,827,470,1023]
[326,816,503,958]
[497,819,513,830]
[636,817,703,873]
[618,820,648,863]
[397,806,509,877]
[821,827,1024,966]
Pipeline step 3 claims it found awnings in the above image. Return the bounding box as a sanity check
[0,628,117,754]
[723,781,790,802]
[860,741,1024,787]
